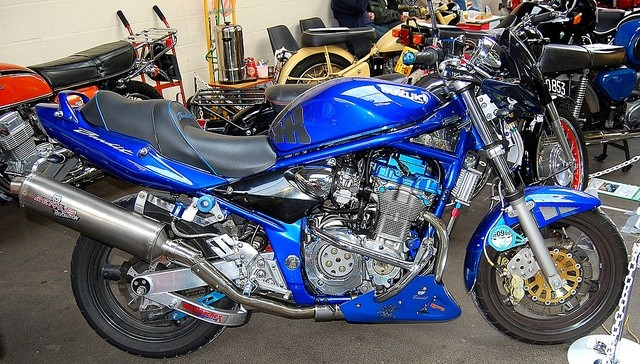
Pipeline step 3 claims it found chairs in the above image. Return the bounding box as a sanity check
[299,17,326,34]
[267,24,299,62]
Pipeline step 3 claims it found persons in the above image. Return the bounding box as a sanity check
[330,0,428,51]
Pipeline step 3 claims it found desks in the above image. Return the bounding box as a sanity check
[208,77,274,115]
[407,10,507,29]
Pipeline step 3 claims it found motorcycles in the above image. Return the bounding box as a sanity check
[280,6,459,85]
[536,12,640,171]
[11,38,627,357]
[0,28,176,201]
[191,33,588,190]
[393,0,599,86]
